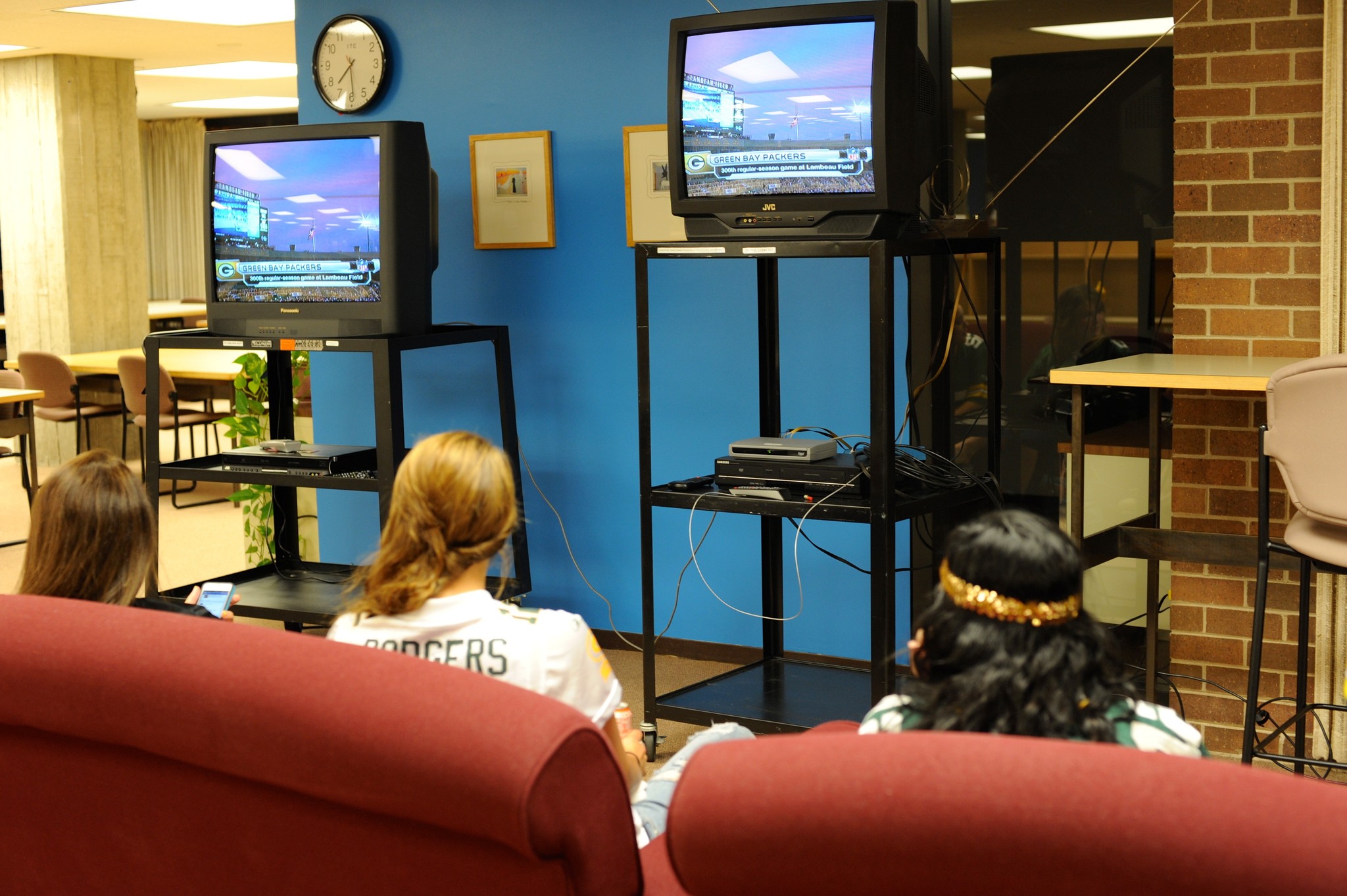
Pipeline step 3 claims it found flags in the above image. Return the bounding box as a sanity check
[308,228,314,239]
[791,115,797,127]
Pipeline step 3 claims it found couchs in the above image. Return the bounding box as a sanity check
[0,597,682,896]
[667,730,1347,896]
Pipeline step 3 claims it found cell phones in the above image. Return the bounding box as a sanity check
[196,581,235,620]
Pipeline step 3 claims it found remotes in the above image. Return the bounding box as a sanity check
[729,486,793,501]
[259,439,301,453]
[667,477,714,491]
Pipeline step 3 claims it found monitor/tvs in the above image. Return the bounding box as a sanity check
[669,0,940,242]
[204,121,439,339]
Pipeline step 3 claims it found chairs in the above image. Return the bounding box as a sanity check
[1242,353,1347,779]
[0,370,34,548]
[18,351,123,492]
[118,355,234,509]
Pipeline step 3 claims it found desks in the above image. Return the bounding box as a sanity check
[147,300,207,333]
[1047,352,1309,730]
[4,348,271,509]
[0,389,45,492]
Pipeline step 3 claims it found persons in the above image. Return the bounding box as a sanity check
[858,510,1211,760]
[327,430,756,851]
[17,450,241,621]
[688,172,875,193]
[218,284,381,301]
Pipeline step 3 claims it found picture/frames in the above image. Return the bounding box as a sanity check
[469,130,555,249]
[623,125,686,246]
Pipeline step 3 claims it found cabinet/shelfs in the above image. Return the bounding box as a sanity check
[139,324,532,631]
[633,240,1159,758]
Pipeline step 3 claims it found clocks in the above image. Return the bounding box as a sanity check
[312,14,393,114]
